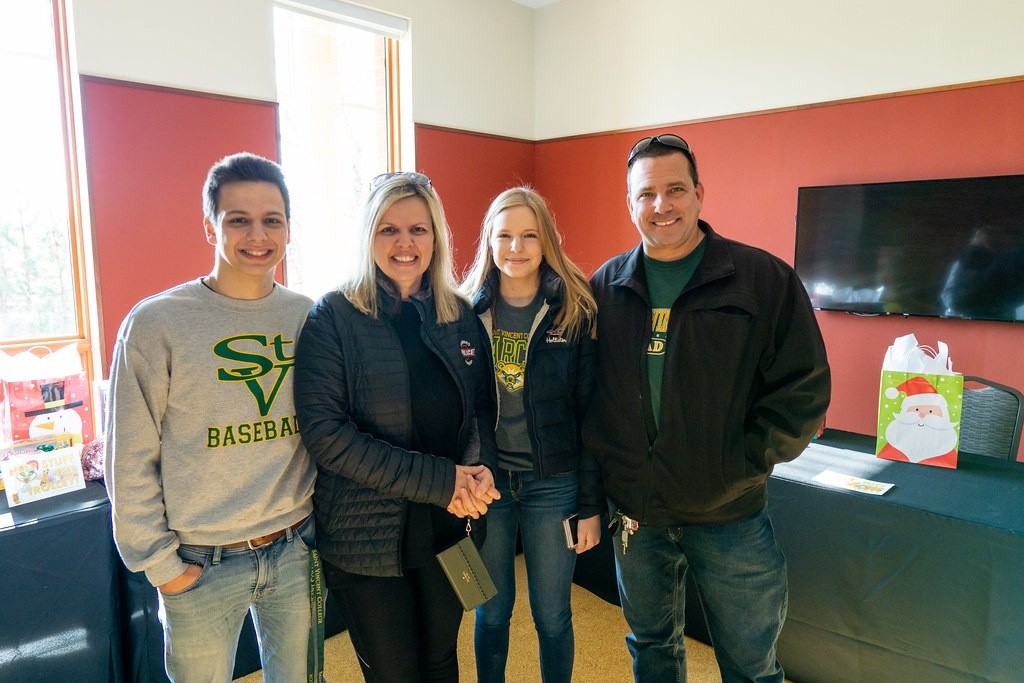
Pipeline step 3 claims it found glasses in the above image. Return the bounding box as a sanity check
[368,171,433,194]
[626,133,692,164]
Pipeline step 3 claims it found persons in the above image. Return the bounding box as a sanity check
[574,137,831,683]
[294,174,500,683]
[103,152,329,683]
[457,188,604,683]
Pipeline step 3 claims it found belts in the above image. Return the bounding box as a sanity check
[214,521,305,553]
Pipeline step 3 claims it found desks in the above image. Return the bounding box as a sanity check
[98,476,348,683]
[570,428,1024,683]
[0,479,128,683]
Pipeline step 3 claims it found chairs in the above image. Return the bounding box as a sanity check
[958,377,1024,461]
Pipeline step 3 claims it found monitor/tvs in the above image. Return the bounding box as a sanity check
[794,175,1024,323]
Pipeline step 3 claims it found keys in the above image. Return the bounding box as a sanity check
[604,508,638,553]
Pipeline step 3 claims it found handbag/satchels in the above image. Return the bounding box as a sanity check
[875,343,964,469]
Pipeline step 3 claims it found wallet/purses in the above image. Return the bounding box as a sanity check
[435,536,499,612]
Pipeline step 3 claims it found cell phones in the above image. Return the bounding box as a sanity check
[562,512,580,550]
